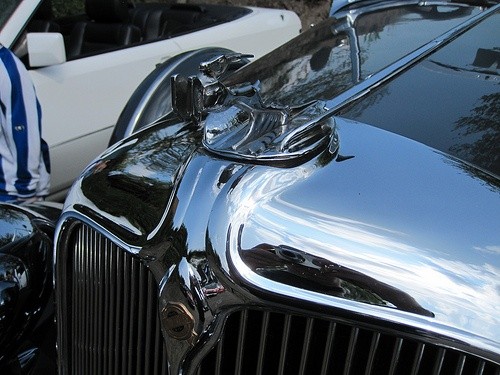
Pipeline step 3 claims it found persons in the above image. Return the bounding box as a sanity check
[242,241,439,320]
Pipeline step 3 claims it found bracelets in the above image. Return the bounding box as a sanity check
[365,277,376,292]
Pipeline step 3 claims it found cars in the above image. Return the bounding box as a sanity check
[48,0,500,375]
[0,0,305,211]
